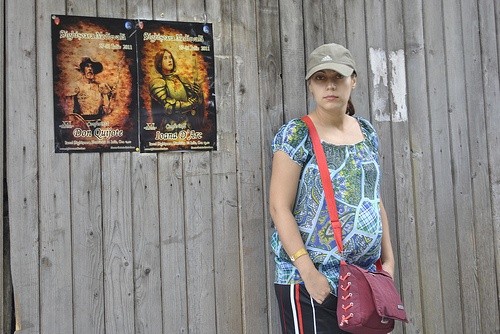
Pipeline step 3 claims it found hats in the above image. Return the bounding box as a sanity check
[305,43,357,78]
[76,56,103,75]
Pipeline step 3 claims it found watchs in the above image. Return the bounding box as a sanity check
[289,248,308,262]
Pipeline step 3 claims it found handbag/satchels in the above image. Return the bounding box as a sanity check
[338,261,408,334]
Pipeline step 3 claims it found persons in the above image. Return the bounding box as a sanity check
[269,43,396,334]
